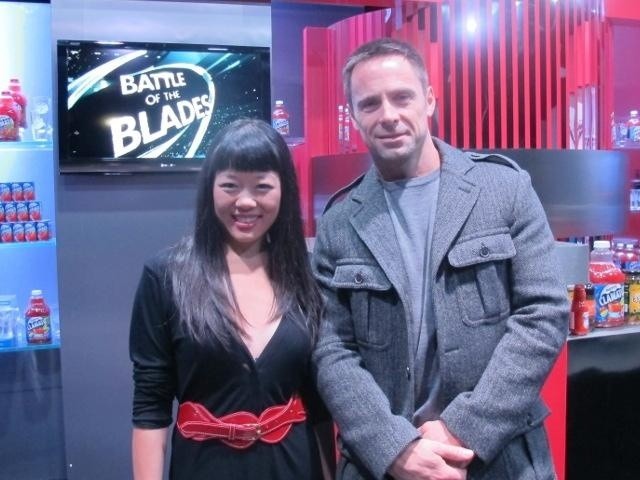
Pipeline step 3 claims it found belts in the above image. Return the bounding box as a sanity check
[174,393,309,451]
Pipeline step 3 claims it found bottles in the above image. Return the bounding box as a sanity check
[566,240,640,336]
[25,290,52,345]
[629,110,640,142]
[272,100,290,137]
[0,78,27,142]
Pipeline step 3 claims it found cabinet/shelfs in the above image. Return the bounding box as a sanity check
[0,0,70,480]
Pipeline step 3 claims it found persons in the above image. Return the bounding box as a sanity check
[307,35,576,479]
[125,115,339,480]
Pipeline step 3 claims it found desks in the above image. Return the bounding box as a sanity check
[538,323,640,480]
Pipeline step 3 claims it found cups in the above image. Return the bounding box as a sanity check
[29,95,52,147]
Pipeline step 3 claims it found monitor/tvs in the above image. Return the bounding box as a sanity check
[56,39,271,175]
